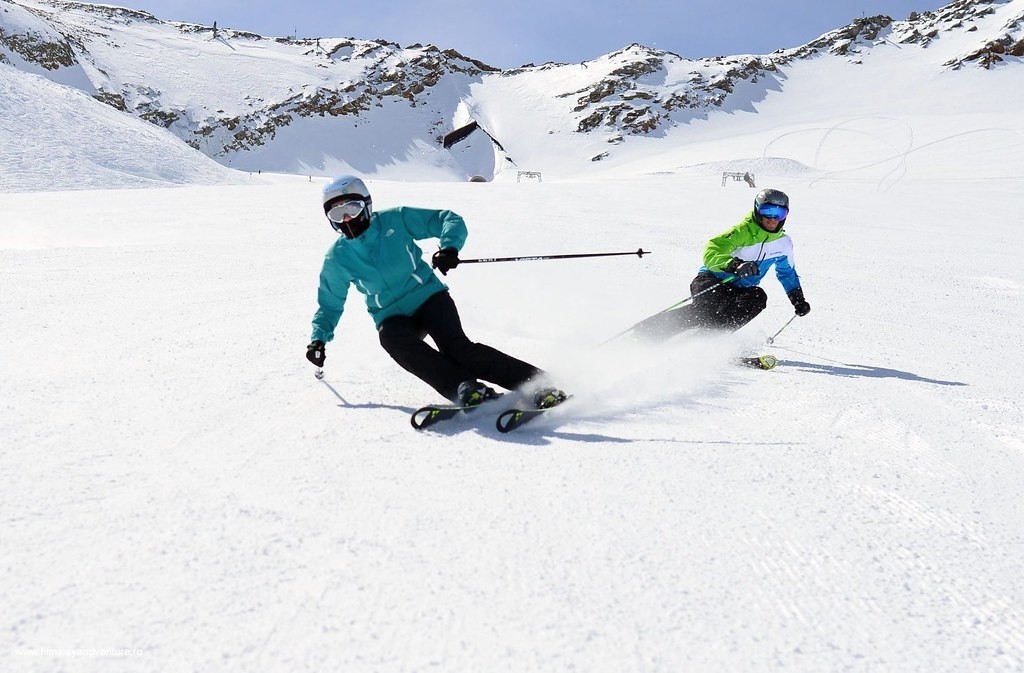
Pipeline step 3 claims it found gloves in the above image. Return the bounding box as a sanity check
[794,301,812,318]
[431,247,462,276]
[306,339,327,368]
[727,256,762,278]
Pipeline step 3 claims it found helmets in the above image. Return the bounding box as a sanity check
[322,174,373,211]
[753,188,790,213]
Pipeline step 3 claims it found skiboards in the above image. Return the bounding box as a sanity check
[410,393,574,433]
[628,334,777,372]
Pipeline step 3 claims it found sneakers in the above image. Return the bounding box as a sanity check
[536,388,568,409]
[458,381,498,408]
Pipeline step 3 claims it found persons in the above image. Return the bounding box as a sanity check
[636,188,809,344]
[306,175,567,407]
[744,172,755,187]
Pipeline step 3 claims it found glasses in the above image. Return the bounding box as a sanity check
[326,200,366,225]
[759,204,789,222]
